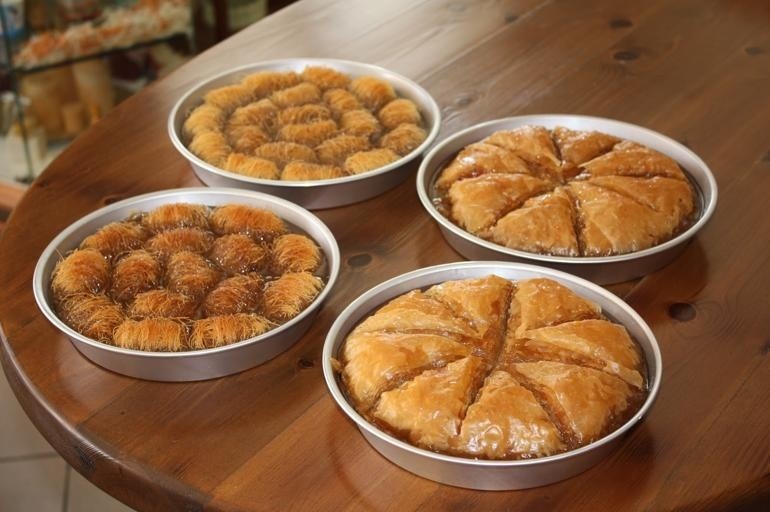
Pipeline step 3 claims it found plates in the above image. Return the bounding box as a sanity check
[166,58,442,212]
[320,260,664,491]
[415,112,718,287]
[30,186,344,384]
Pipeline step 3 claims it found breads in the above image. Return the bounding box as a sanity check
[332,273,651,459]
[180,67,423,181]
[49,203,324,352]
[434,124,697,257]
